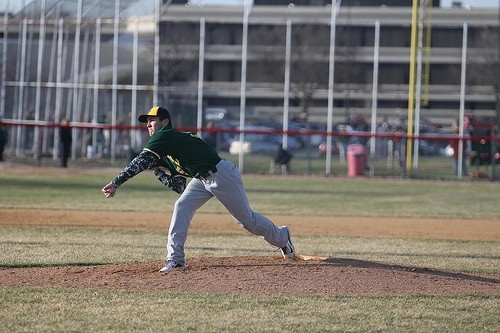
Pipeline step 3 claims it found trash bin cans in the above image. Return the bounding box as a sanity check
[347,144,366,177]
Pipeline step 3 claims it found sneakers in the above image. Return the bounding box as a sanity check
[159,261,185,272]
[280,226,295,259]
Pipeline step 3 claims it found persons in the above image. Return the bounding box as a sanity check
[102,106,296,273]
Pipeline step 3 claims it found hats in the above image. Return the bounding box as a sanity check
[138,106,171,123]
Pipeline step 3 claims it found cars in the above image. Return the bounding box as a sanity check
[199,108,474,158]
